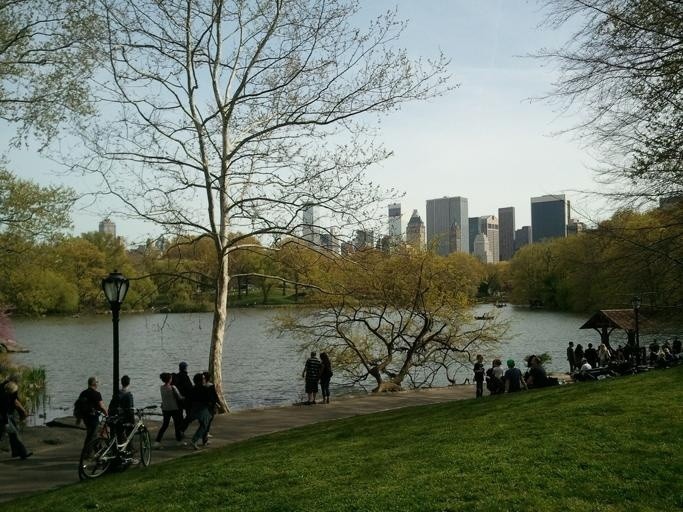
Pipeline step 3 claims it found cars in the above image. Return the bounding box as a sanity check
[76,404,157,480]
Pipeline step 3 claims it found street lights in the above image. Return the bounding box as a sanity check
[630,294,642,373]
[100,267,130,396]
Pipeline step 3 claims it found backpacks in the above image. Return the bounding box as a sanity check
[74,396,90,420]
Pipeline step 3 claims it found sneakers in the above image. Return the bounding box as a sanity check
[20,453,33,460]
[152,433,213,450]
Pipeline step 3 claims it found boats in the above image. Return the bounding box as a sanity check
[493,304,507,308]
[473,315,495,321]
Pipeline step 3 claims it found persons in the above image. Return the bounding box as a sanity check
[300,351,320,404]
[187,372,213,450]
[567,335,683,379]
[74,374,109,469]
[0,368,33,459]
[472,355,486,398]
[151,372,187,449]
[201,369,223,439]
[316,353,333,404]
[109,375,138,455]
[485,354,547,394]
[170,361,194,438]
